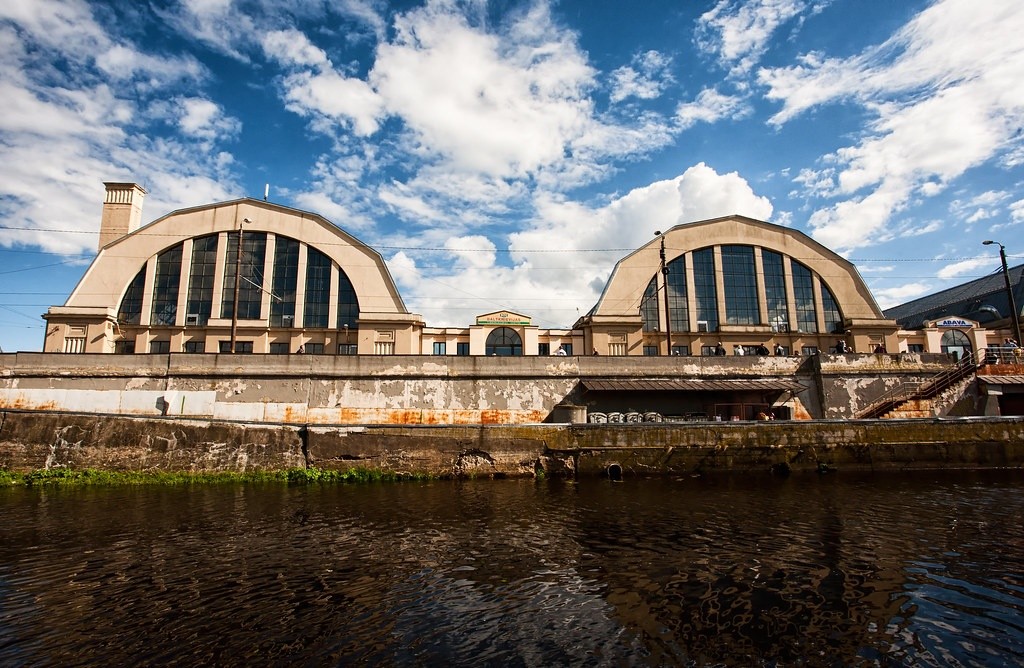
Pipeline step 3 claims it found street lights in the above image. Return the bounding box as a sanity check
[654,231,673,356]
[228,219,252,353]
[982,240,1023,349]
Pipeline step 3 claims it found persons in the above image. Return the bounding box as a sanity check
[557,345,567,355]
[737,344,745,356]
[962,348,972,367]
[757,343,770,355]
[714,342,726,356]
[674,350,680,356]
[793,350,801,356]
[984,338,1019,364]
[775,343,784,356]
[874,343,884,353]
[592,348,599,356]
[297,345,304,354]
